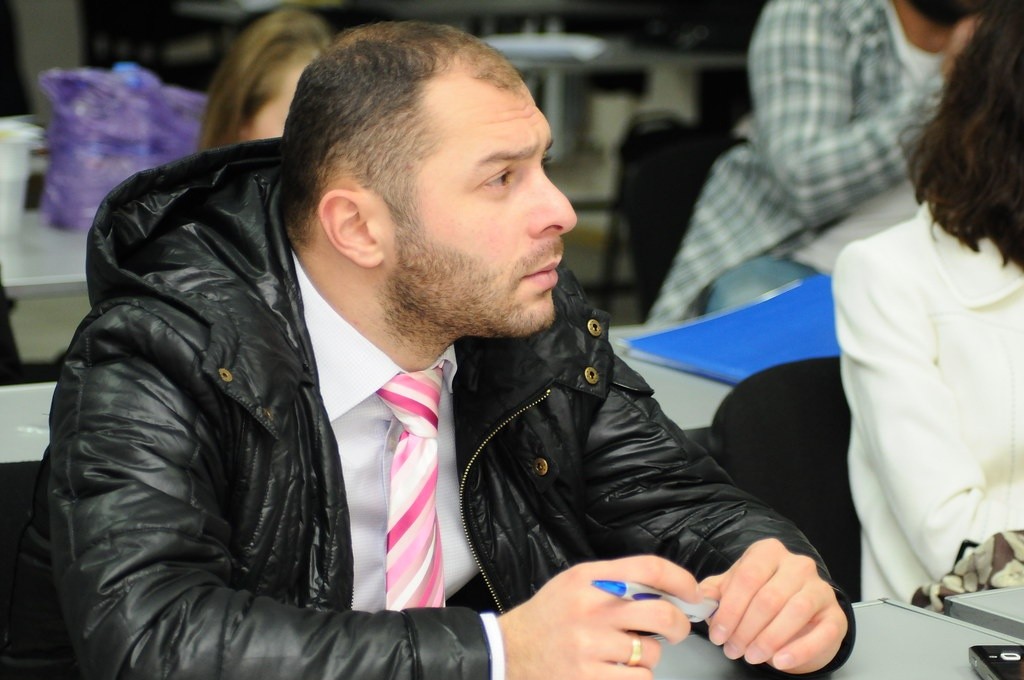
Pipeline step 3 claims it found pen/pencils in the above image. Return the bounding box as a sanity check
[594,578,720,629]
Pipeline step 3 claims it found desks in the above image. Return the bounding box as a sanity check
[610,322,1024,680]
[0,212,88,460]
[501,54,746,161]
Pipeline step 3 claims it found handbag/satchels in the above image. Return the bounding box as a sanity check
[37,62,210,231]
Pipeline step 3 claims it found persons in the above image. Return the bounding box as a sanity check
[830,1,1024,603]
[200,6,330,153]
[1,18,855,680]
[645,1,997,328]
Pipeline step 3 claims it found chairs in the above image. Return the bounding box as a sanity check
[625,119,747,324]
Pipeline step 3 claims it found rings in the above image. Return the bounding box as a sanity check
[627,634,641,665]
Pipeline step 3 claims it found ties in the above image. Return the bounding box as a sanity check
[374,360,446,611]
